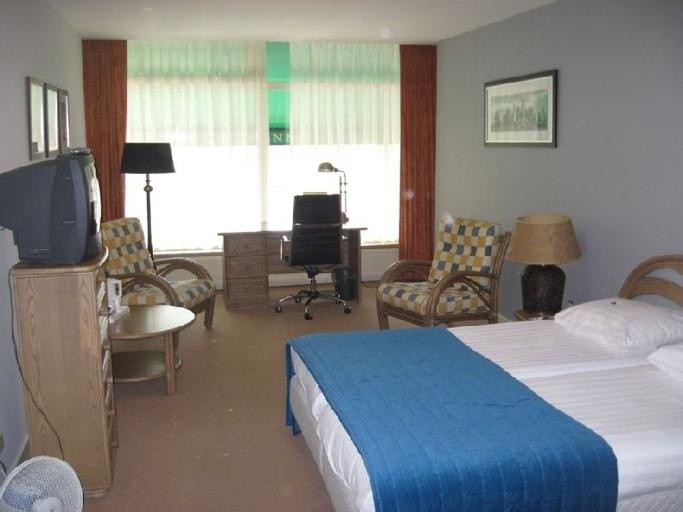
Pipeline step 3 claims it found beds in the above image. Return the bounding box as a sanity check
[282,256,683,512]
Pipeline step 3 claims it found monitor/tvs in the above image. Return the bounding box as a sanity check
[0,154,102,265]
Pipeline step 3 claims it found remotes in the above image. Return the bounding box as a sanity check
[81,149,91,155]
[70,147,85,154]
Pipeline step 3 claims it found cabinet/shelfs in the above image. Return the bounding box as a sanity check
[11,248,120,500]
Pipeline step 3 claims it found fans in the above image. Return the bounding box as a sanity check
[0,456,84,512]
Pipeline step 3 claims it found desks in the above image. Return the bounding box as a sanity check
[217,226,366,310]
[108,306,195,396]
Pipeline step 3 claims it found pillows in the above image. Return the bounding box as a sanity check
[650,343,683,381]
[551,298,683,352]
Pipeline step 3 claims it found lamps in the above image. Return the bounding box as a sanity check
[318,161,348,214]
[506,212,579,311]
[119,142,176,272]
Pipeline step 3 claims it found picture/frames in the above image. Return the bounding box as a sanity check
[482,69,554,150]
[25,75,46,161]
[57,87,69,153]
[43,81,59,158]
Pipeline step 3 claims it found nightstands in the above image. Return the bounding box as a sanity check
[511,310,548,322]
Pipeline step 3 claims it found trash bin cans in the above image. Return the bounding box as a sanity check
[335,268,355,300]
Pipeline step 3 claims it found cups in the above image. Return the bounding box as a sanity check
[108,300,116,315]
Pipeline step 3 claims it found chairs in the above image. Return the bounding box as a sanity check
[274,192,350,320]
[99,216,216,349]
[373,214,512,329]
